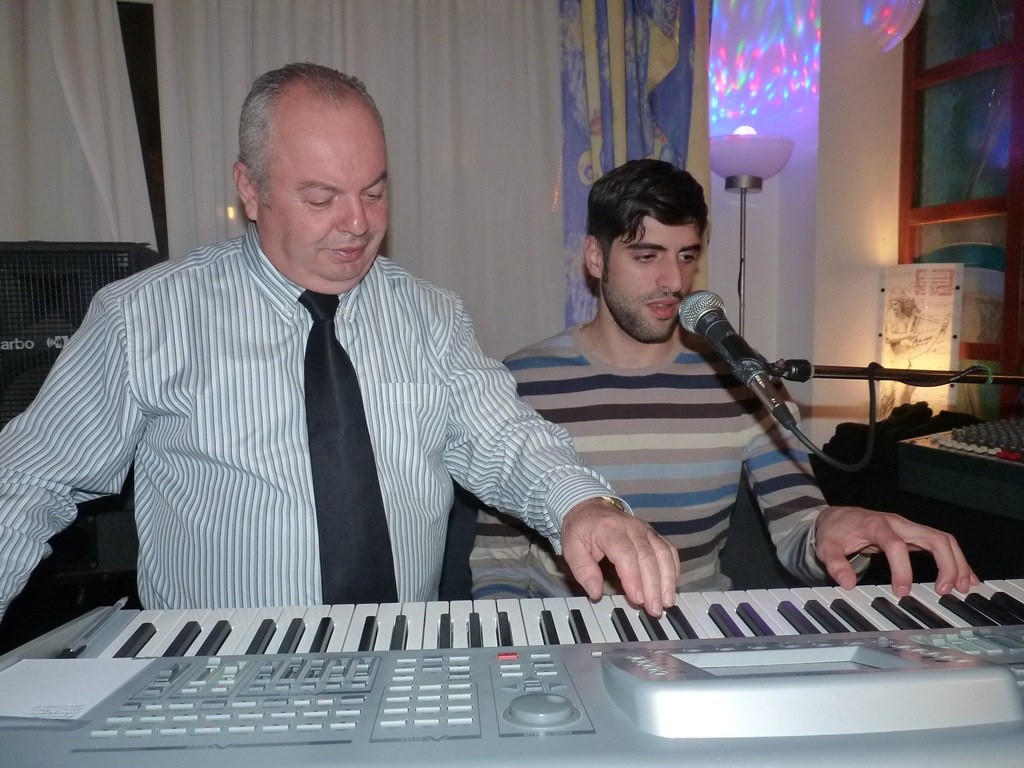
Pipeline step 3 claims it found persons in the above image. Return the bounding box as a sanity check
[1,60,685,620]
[469,161,981,599]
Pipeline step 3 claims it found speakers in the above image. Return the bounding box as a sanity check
[0,240,168,658]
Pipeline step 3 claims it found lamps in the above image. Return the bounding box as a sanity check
[707,126,796,338]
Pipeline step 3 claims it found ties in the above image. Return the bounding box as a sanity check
[297,291,399,605]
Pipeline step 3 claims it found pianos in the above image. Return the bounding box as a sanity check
[1,576,1024,768]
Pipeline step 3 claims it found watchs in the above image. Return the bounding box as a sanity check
[599,495,622,509]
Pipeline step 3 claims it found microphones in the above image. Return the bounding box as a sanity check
[679,289,798,429]
